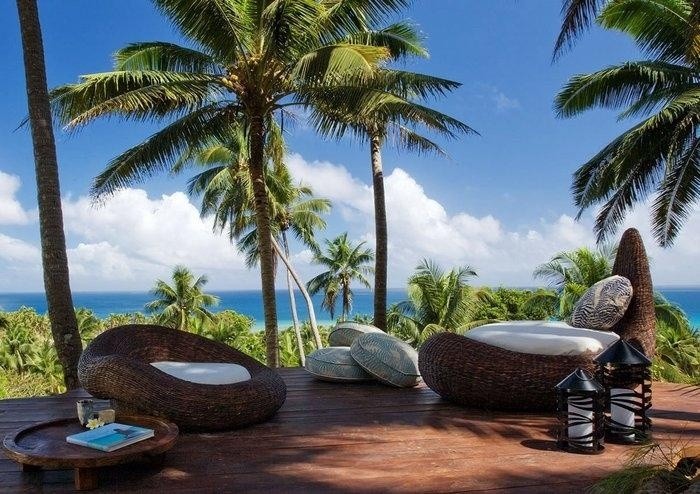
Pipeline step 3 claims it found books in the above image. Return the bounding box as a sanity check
[66,422,155,451]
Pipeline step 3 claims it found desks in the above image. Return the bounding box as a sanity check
[4,410,179,494]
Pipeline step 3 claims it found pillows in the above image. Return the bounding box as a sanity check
[572,275,633,329]
[350,333,422,389]
[328,322,386,346]
[305,347,377,381]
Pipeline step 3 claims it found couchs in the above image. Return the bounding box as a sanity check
[76,323,287,430]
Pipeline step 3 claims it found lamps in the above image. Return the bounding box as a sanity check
[593,338,653,446]
[555,367,606,455]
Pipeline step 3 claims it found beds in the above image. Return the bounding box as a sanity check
[417,228,655,405]
